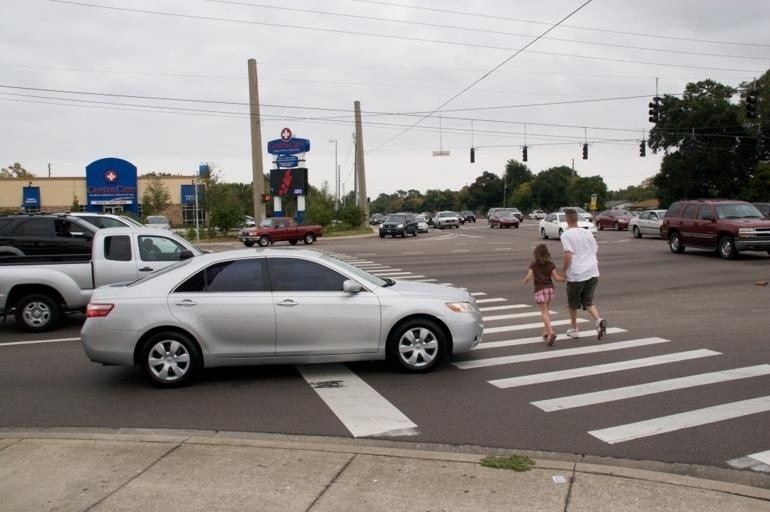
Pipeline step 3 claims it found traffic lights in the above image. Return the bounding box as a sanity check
[639,143,646,157]
[582,146,588,159]
[649,102,658,122]
[522,148,527,162]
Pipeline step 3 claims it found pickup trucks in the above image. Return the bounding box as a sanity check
[238,217,324,247]
[1,226,205,333]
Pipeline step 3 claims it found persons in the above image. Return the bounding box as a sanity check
[62,221,74,237]
[142,238,162,259]
[560,209,607,341]
[273,219,285,227]
[521,243,567,345]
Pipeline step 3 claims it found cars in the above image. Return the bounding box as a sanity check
[628,210,667,239]
[595,210,634,231]
[82,248,486,388]
[487,208,524,229]
[528,210,546,219]
[433,210,476,229]
[539,212,597,239]
[559,207,593,221]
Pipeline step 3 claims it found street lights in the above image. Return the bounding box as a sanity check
[329,139,338,202]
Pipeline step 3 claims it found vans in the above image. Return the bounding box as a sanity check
[662,200,770,260]
[1,211,172,255]
[369,212,432,239]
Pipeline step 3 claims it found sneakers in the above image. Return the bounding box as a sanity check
[543,331,556,346]
[595,319,606,340]
[567,328,578,338]
